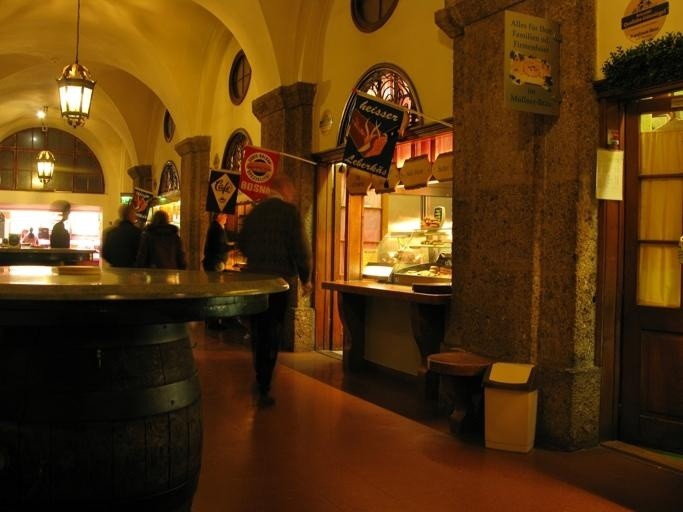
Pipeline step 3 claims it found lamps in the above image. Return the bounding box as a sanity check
[36,122,55,185]
[58,0,98,130]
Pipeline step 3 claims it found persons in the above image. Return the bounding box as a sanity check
[135,210,188,271]
[48,199,72,248]
[237,174,313,409]
[100,203,140,267]
[200,212,238,329]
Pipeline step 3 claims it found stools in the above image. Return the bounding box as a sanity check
[427,348,495,439]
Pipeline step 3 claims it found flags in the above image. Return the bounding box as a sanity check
[204,171,239,215]
[341,95,403,178]
[129,191,151,223]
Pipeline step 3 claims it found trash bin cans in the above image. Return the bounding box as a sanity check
[482,361,541,454]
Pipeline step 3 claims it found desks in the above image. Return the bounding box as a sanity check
[1,247,292,511]
